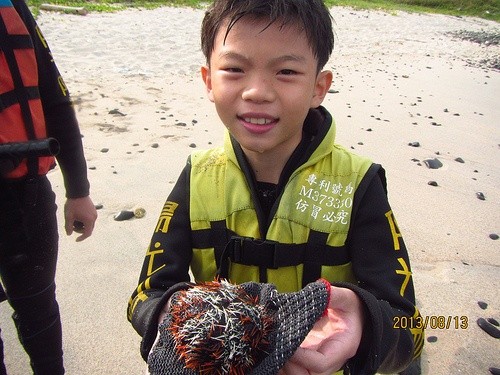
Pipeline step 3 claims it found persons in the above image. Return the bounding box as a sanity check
[124,0,426,373]
[0,0,98,374]
